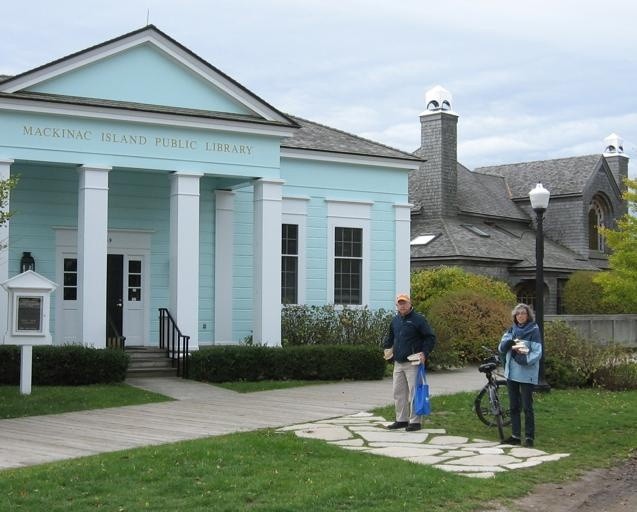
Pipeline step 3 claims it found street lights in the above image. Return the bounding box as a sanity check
[527,180,551,393]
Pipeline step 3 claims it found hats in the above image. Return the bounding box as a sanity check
[396,293,410,303]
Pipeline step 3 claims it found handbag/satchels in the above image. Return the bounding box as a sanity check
[413,362,430,416]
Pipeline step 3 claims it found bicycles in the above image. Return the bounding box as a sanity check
[472,345,523,440]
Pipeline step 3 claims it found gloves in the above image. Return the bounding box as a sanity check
[511,349,518,359]
[506,339,515,349]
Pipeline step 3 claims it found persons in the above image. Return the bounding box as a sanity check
[498,302,543,448]
[383,295,436,432]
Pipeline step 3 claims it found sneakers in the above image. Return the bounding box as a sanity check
[500,436,521,445]
[405,422,421,432]
[523,439,533,448]
[387,421,408,430]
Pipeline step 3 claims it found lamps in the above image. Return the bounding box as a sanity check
[20,250,35,273]
